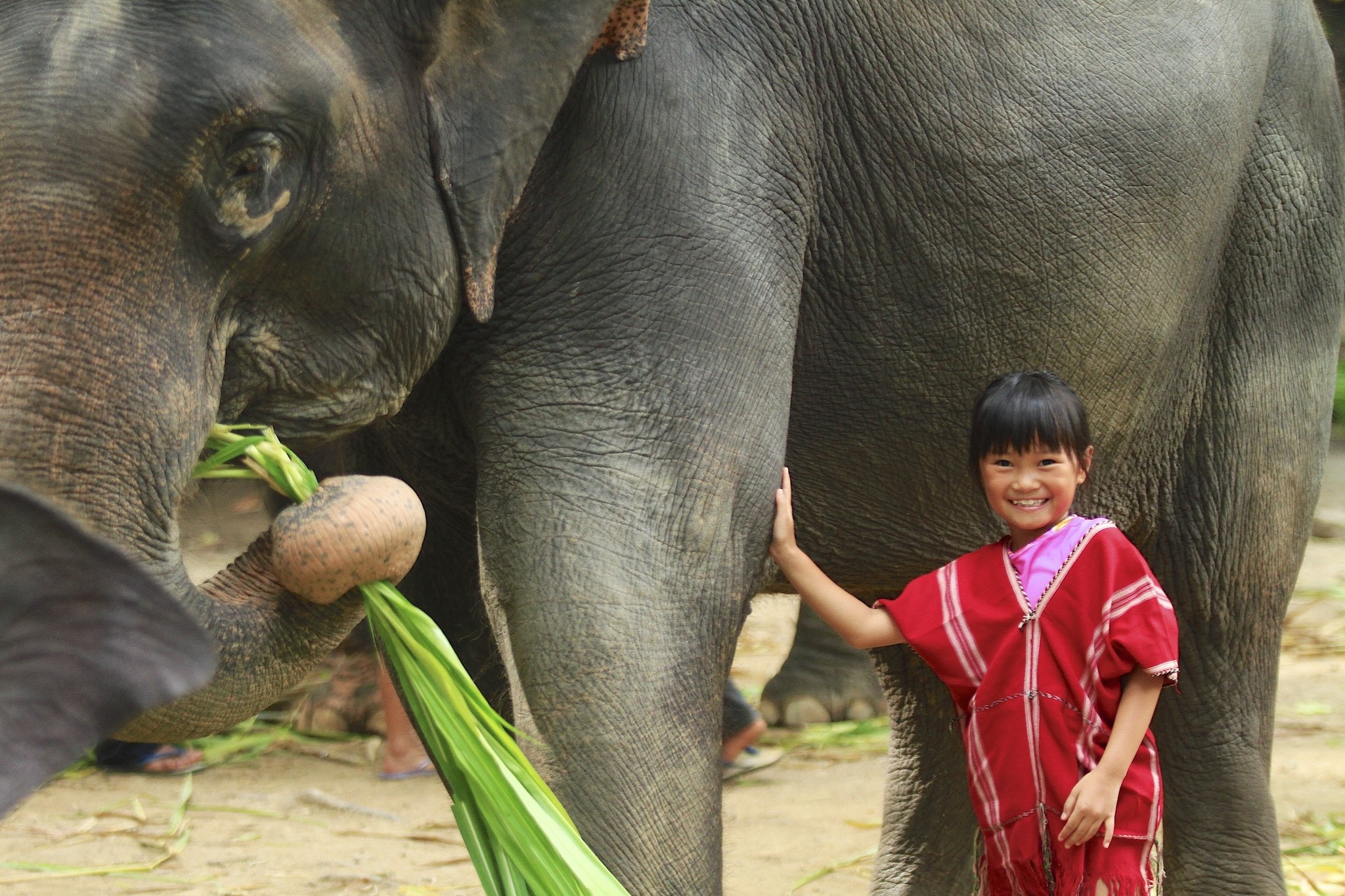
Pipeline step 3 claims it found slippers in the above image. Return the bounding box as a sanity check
[722,746,784,782]
[380,757,437,782]
[136,742,205,775]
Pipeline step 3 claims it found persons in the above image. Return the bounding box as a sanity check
[768,373,1179,896]
[379,655,437,775]
[143,741,204,772]
[722,679,766,769]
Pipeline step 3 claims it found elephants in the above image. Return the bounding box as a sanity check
[1,0,1345,896]
